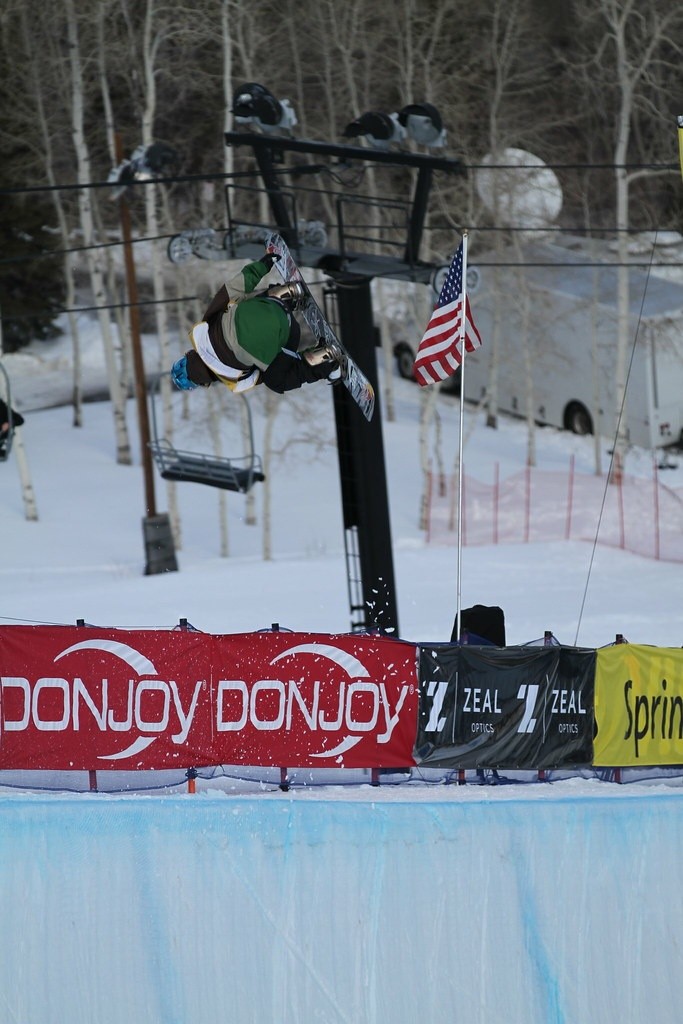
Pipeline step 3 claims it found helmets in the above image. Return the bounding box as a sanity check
[171,355,199,392]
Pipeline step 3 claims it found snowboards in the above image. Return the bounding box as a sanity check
[265,234,376,423]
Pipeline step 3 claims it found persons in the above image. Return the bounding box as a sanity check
[172,252,342,394]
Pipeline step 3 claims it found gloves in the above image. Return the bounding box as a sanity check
[259,252,282,273]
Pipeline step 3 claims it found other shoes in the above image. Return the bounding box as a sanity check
[266,282,306,312]
[302,342,348,385]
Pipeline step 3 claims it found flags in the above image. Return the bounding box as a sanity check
[414,240,482,387]
